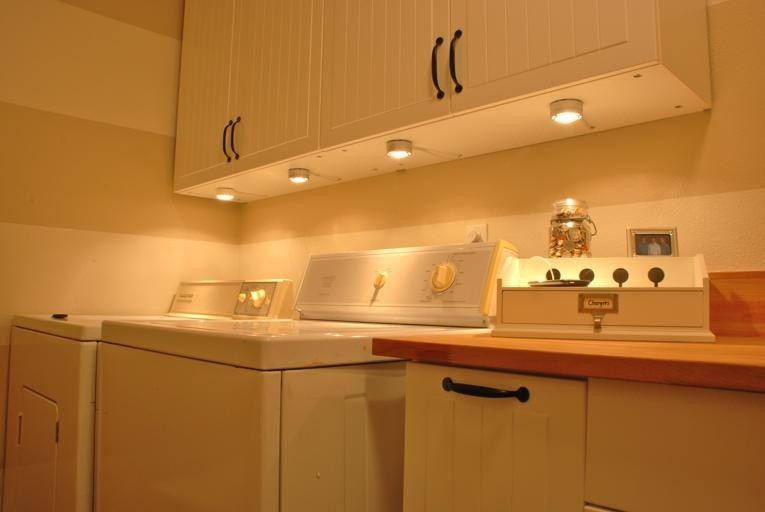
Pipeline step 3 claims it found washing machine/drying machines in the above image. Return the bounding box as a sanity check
[2,280,298,512]
[93,239,517,511]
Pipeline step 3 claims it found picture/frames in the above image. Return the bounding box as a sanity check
[626,226,679,257]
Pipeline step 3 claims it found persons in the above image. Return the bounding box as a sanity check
[647,237,662,255]
[639,236,648,255]
[659,237,671,255]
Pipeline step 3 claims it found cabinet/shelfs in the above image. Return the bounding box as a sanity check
[172,0,342,203]
[372,270,765,511]
[318,0,712,182]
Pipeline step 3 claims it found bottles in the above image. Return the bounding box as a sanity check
[549,198,595,257]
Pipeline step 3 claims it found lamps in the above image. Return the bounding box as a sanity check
[215,188,234,200]
[288,168,309,183]
[550,99,583,124]
[386,140,411,158]
